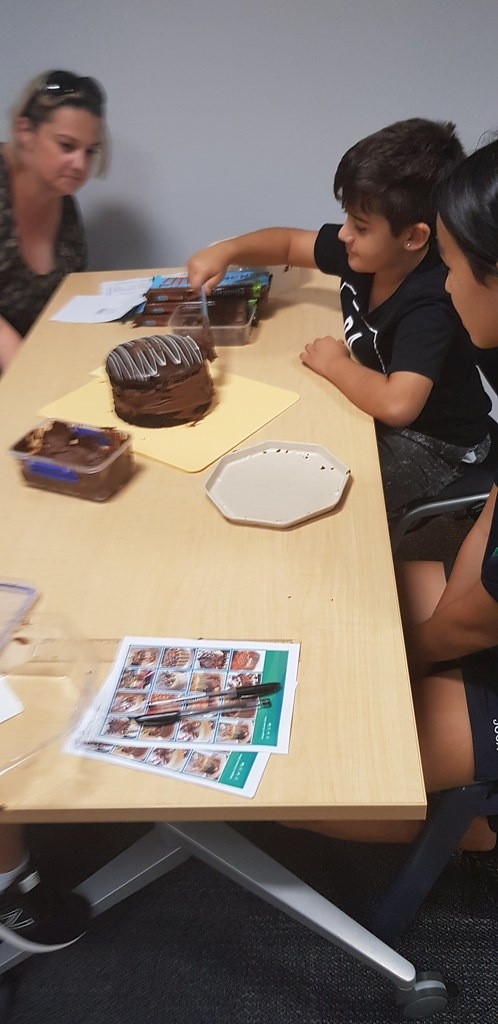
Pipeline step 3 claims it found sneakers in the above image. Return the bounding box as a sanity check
[2,854,92,953]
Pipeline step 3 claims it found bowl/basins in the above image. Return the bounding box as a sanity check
[168,303,258,347]
[6,419,133,501]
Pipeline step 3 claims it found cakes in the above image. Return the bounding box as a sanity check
[105,333,216,428]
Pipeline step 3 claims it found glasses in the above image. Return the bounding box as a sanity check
[18,76,105,117]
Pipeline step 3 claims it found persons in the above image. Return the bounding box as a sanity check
[0,69,106,381]
[270,139,497,853]
[188,117,492,519]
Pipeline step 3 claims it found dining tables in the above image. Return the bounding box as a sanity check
[0,265,451,1021]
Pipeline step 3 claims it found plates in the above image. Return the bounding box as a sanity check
[204,440,350,529]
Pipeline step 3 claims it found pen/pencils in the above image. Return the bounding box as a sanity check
[134,697,272,726]
[147,682,282,708]
